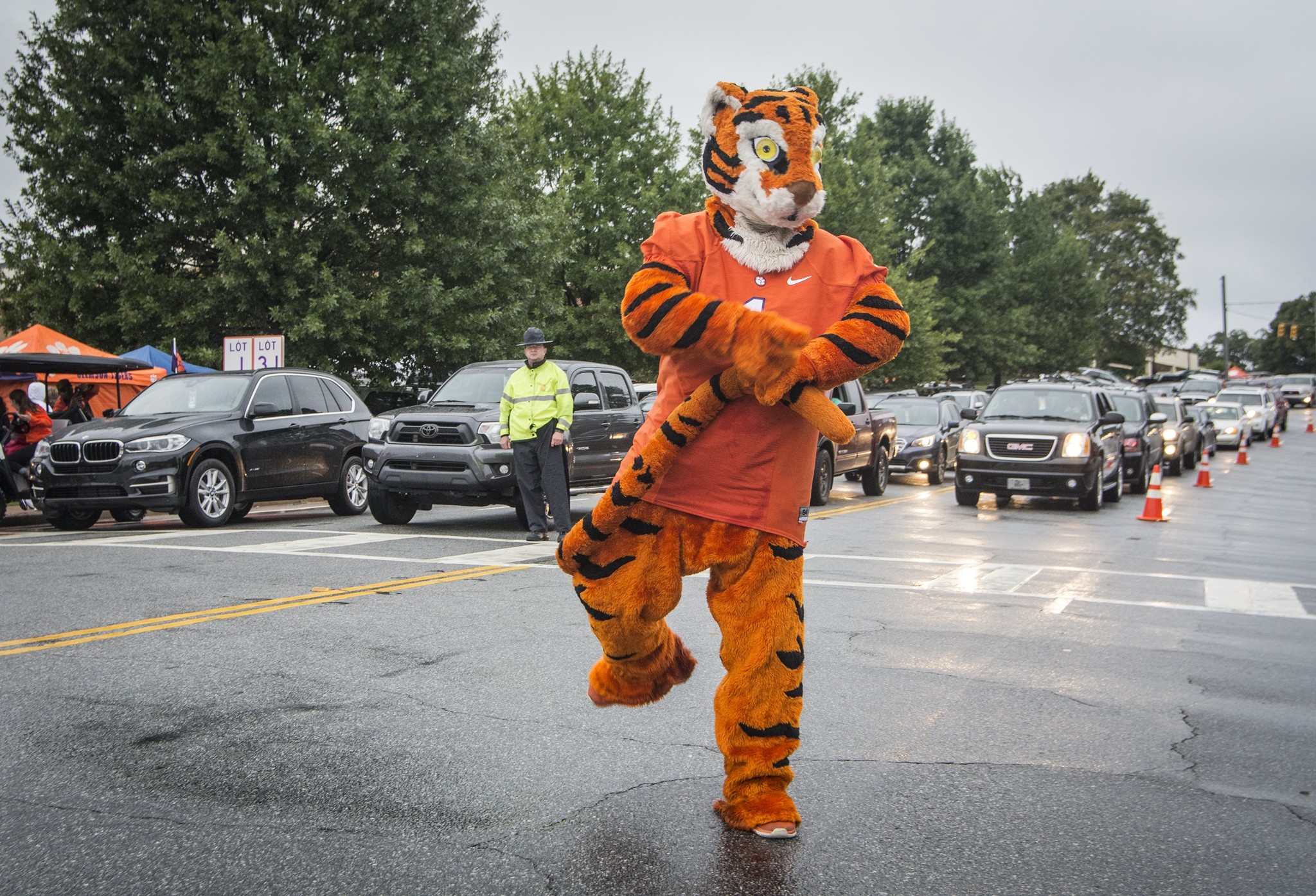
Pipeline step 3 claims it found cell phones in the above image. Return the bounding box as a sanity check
[74,386,82,400]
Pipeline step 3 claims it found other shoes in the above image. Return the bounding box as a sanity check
[23,468,35,481]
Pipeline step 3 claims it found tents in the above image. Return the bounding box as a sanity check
[0,323,166,417]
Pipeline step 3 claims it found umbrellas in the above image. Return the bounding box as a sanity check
[118,345,219,374]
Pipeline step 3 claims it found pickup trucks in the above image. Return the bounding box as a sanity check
[812,375,895,506]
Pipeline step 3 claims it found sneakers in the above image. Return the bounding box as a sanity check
[18,498,27,510]
[587,660,687,708]
[25,499,35,509]
[557,531,569,543]
[752,820,798,838]
[526,530,550,541]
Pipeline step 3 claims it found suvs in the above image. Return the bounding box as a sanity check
[1100,385,1168,492]
[29,365,374,529]
[872,394,963,486]
[360,360,646,525]
[956,382,1125,509]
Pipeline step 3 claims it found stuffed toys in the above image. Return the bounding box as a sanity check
[556,77,913,838]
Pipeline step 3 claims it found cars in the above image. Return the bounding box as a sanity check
[931,391,990,418]
[1154,396,1198,477]
[1041,369,1316,445]
[1189,406,1216,460]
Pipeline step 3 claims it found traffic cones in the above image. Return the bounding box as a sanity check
[1305,411,1315,433]
[1191,451,1214,488]
[1266,424,1281,448]
[1231,435,1250,465]
[1137,464,1171,522]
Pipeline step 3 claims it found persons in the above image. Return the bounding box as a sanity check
[6,389,53,479]
[0,381,60,443]
[499,326,574,544]
[18,381,47,511]
[52,379,99,420]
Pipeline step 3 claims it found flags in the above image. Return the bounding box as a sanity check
[171,342,185,372]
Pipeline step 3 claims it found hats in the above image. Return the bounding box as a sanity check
[56,379,72,392]
[515,327,553,347]
